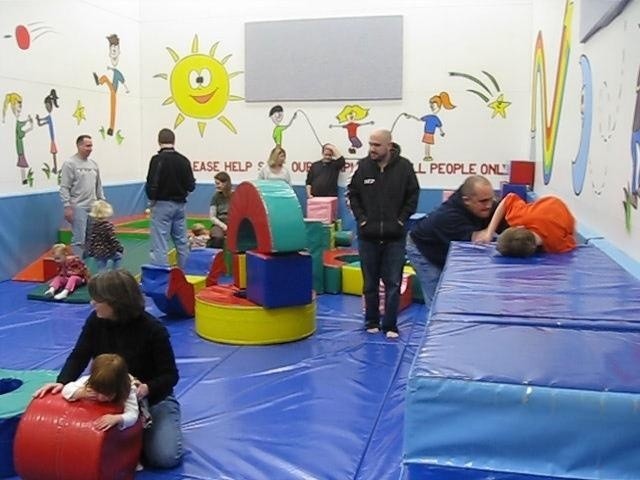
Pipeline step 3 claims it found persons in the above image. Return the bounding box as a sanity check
[59,134,105,259]
[469,190,577,258]
[62,351,153,474]
[84,199,124,272]
[41,242,89,300]
[188,222,211,248]
[258,146,291,186]
[305,144,346,199]
[144,128,197,268]
[208,171,234,248]
[403,174,511,323]
[342,129,420,339]
[31,268,184,470]
